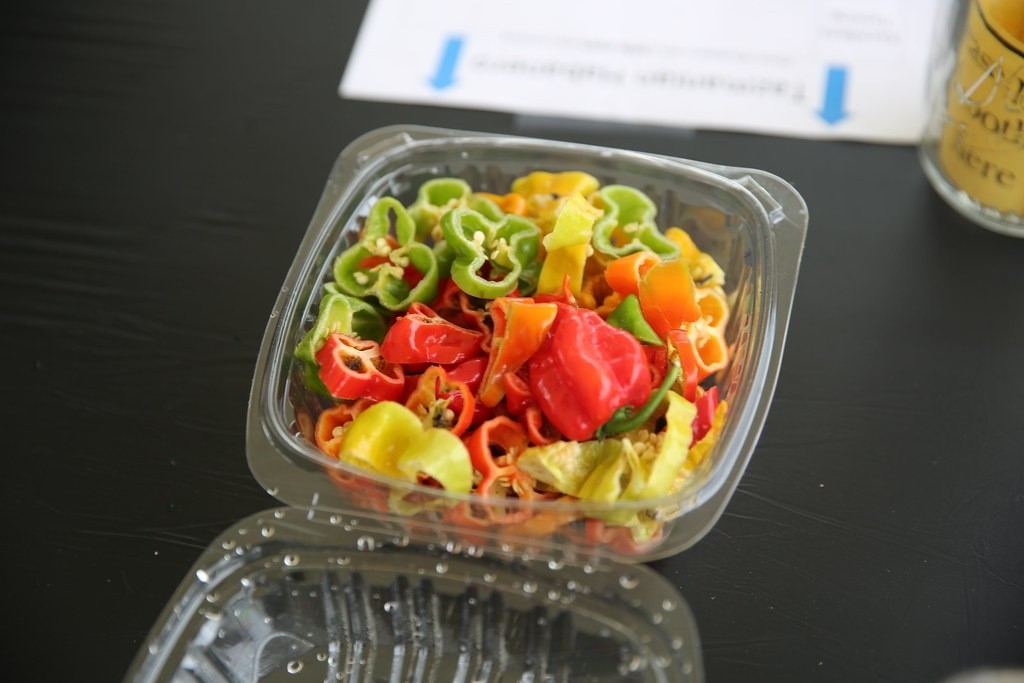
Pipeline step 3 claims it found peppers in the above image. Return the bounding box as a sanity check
[293,167,732,556]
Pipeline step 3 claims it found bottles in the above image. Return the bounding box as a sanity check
[917,0,1024,239]
